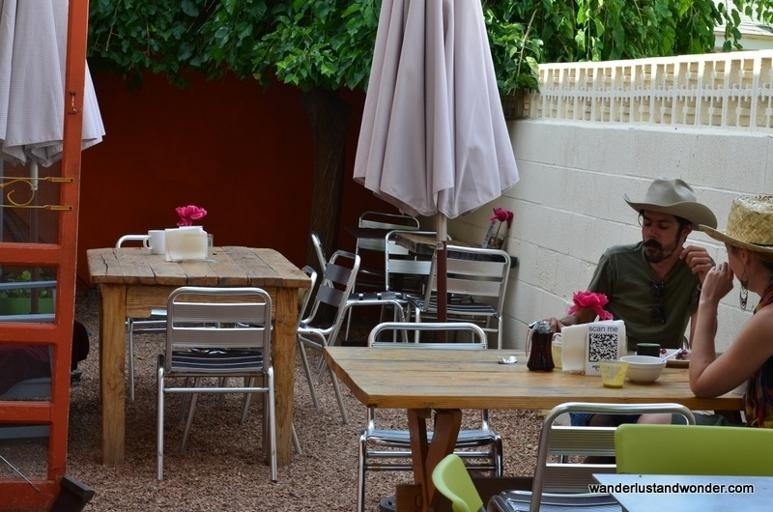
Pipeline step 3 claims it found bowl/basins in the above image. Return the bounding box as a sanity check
[619,354,666,383]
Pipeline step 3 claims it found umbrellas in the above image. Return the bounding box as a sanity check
[0,0,107,312]
[352,1,520,342]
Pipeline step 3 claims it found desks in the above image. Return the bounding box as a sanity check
[88,247,311,467]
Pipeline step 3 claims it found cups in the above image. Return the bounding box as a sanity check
[598,360,629,387]
[142,229,164,255]
[551,333,563,369]
[206,235,214,256]
[636,342,660,356]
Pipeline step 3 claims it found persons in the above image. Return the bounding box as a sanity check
[546,180,718,462]
[600,192,772,462]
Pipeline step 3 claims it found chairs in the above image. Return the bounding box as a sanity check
[116,234,221,403]
[154,211,772,512]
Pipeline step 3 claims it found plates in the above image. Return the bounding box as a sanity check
[660,348,690,365]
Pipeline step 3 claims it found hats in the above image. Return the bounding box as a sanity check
[624,179,717,229]
[700,194,773,252]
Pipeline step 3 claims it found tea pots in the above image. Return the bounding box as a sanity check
[525,319,556,370]
